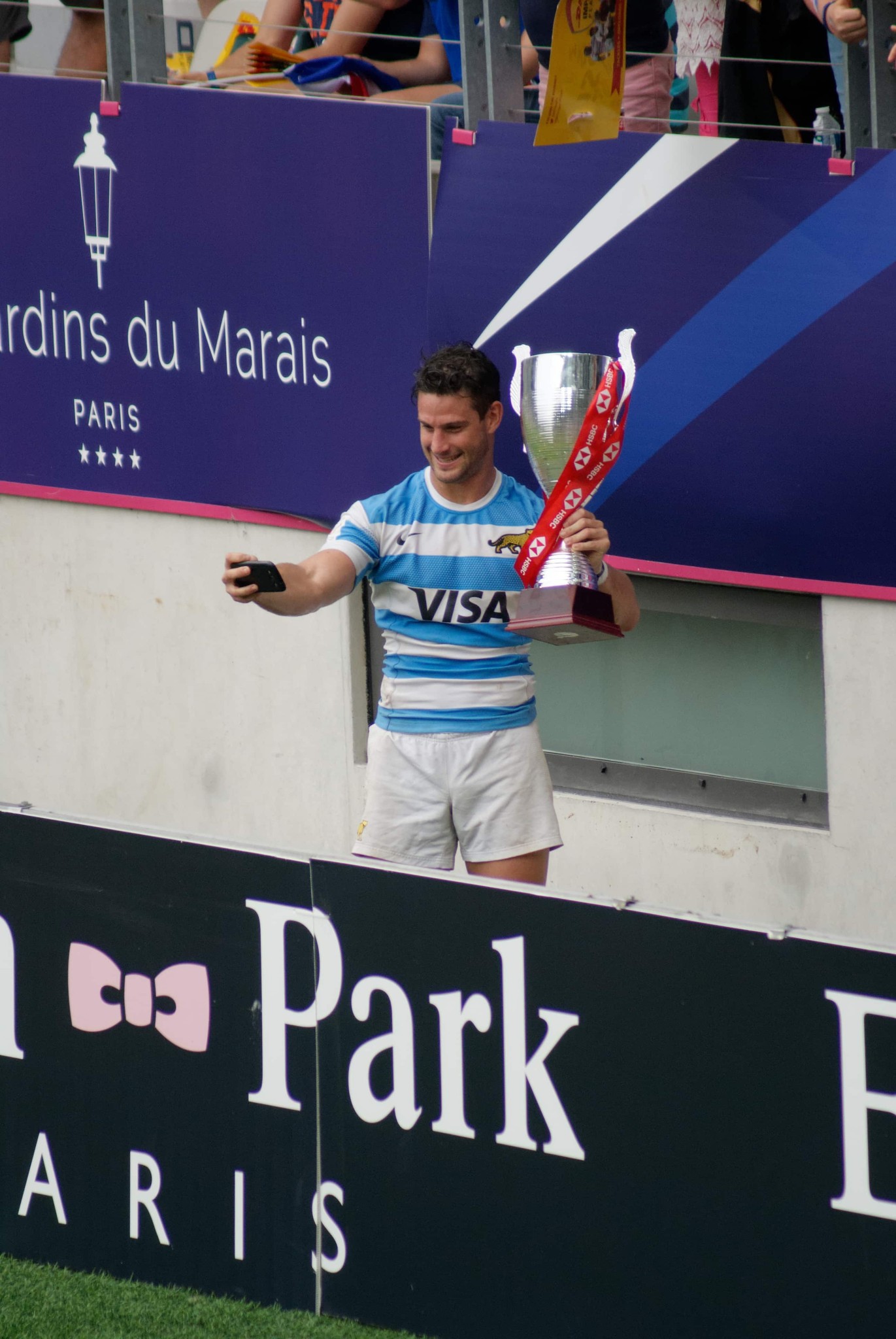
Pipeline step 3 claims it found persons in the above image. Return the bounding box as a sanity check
[0,0,896,150]
[221,347,640,886]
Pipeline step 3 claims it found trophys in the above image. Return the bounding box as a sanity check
[506,328,638,649]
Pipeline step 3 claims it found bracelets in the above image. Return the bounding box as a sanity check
[596,558,609,586]
[822,0,838,35]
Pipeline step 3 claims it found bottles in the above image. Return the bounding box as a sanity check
[813,106,841,159]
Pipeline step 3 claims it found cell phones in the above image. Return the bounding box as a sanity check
[231,560,286,592]
